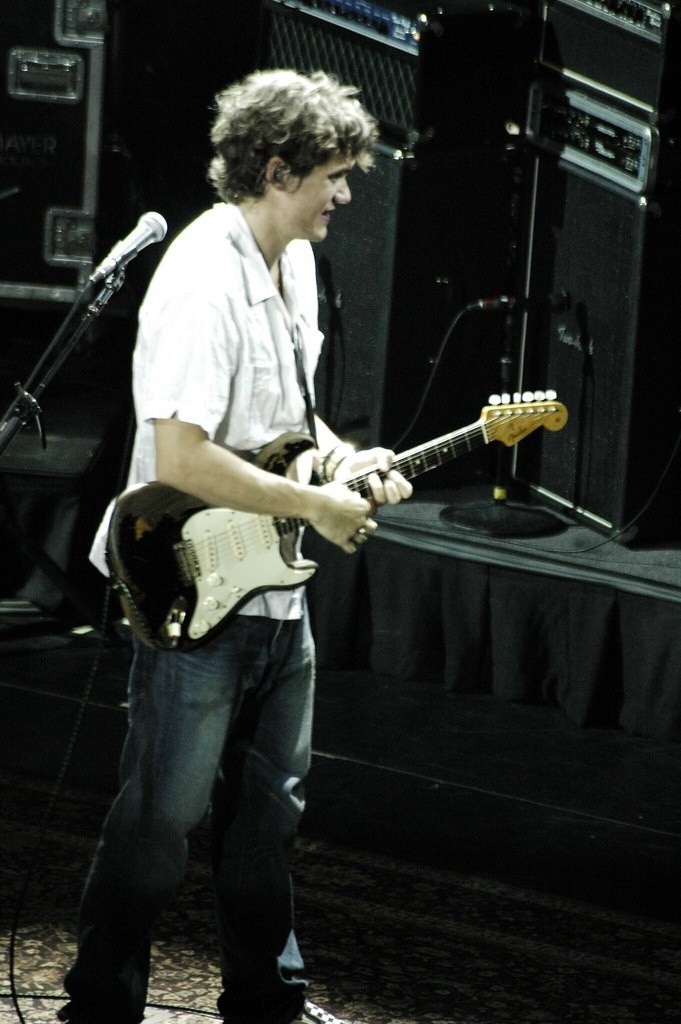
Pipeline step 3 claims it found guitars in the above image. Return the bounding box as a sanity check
[103,389,570,655]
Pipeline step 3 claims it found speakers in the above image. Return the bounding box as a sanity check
[253,0,681,546]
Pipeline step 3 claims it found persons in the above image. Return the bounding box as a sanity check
[56,66,414,1024]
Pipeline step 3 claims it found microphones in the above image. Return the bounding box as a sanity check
[88,211,167,284]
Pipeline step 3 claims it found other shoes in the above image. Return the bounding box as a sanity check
[291,997,345,1024]
